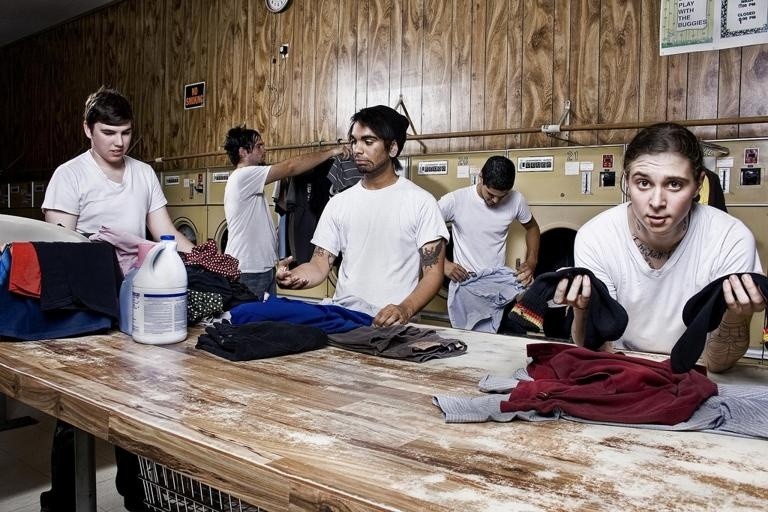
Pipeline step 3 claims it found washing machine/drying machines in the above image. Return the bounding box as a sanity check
[1,134,767,354]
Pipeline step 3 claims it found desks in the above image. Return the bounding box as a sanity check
[0,317,768,512]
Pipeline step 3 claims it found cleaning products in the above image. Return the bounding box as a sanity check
[118,235,189,348]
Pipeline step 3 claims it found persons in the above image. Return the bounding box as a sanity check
[38,85,201,512]
[274,103,450,331]
[437,155,540,333]
[222,125,354,306]
[551,121,768,374]
